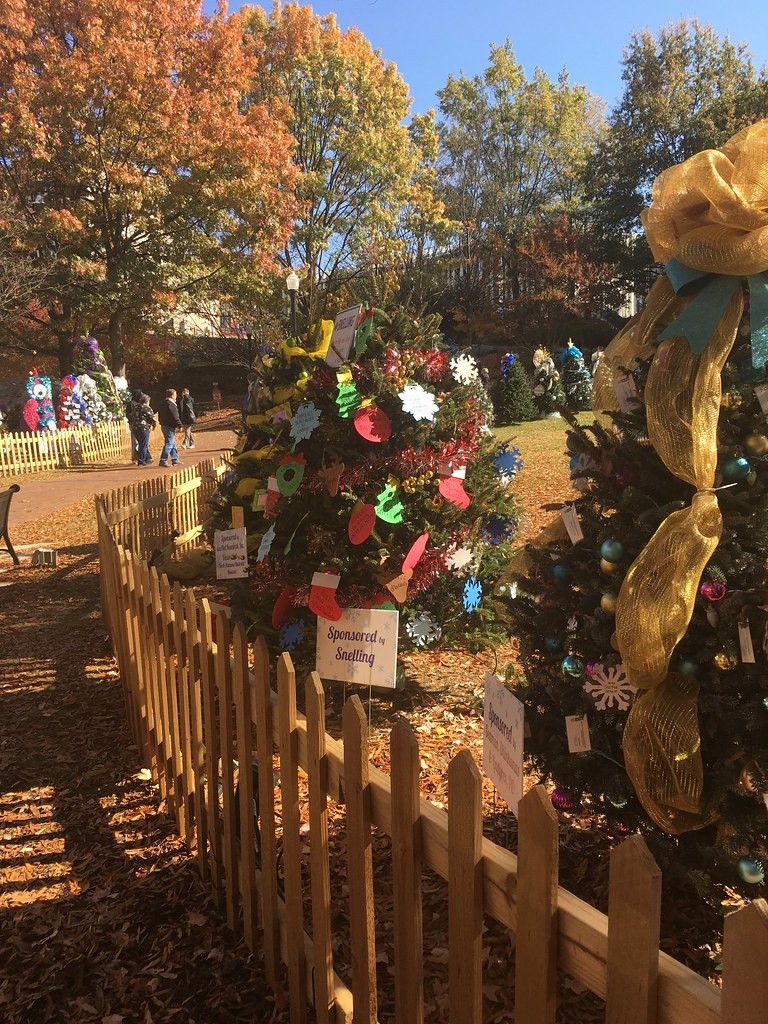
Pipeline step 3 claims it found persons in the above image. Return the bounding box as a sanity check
[127,390,156,466]
[178,388,197,450]
[158,390,184,468]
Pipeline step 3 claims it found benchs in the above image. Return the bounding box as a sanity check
[0,484,22,566]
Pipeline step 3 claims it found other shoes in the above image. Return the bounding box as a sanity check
[188,444,195,449]
[180,444,187,450]
[137,462,147,467]
[158,461,172,467]
[172,460,184,466]
